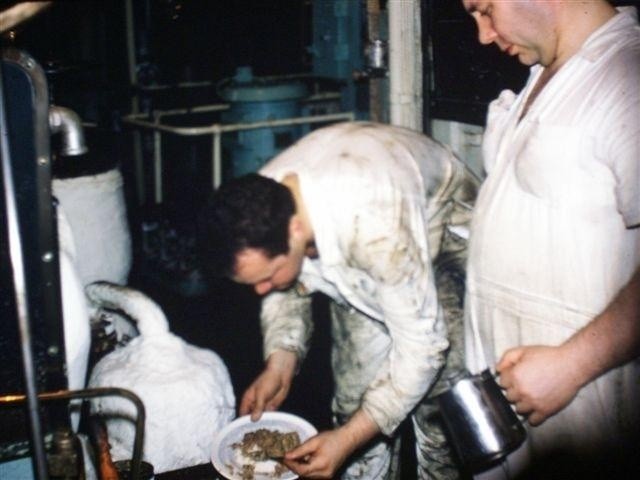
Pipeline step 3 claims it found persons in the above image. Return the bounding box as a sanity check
[460,0,640,479]
[200,120,483,480]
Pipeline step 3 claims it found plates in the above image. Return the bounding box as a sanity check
[211,410,319,480]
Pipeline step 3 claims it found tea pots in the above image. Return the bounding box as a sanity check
[427,365,528,477]
